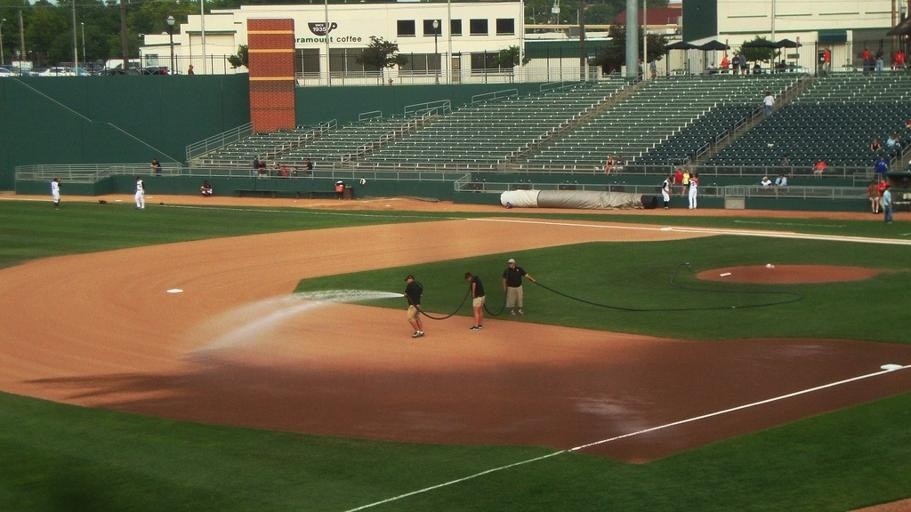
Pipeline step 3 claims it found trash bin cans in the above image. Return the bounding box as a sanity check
[344,184,353,199]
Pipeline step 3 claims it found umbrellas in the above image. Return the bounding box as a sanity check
[665,42,700,73]
[771,39,804,63]
[742,39,777,66]
[699,40,731,67]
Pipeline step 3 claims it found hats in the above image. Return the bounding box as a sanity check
[405,275,415,281]
[508,258,515,264]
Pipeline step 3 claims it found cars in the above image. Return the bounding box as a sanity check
[667,60,814,76]
[1,60,188,79]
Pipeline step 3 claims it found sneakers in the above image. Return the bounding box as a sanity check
[470,325,481,331]
[510,311,524,316]
[412,331,423,337]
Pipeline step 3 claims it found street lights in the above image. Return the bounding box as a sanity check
[795,35,802,75]
[80,22,88,69]
[165,14,178,77]
[430,18,443,84]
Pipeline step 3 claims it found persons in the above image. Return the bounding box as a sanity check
[776,173,788,187]
[673,169,683,193]
[866,120,911,224]
[704,46,911,79]
[681,169,691,195]
[465,272,487,333]
[762,176,773,187]
[199,179,213,196]
[151,158,162,178]
[503,258,537,317]
[134,175,146,212]
[617,154,627,172]
[253,156,314,180]
[660,174,672,209]
[604,154,616,176]
[405,274,427,337]
[335,179,344,202]
[688,172,698,209]
[763,92,777,118]
[813,158,828,181]
[50,176,62,208]
